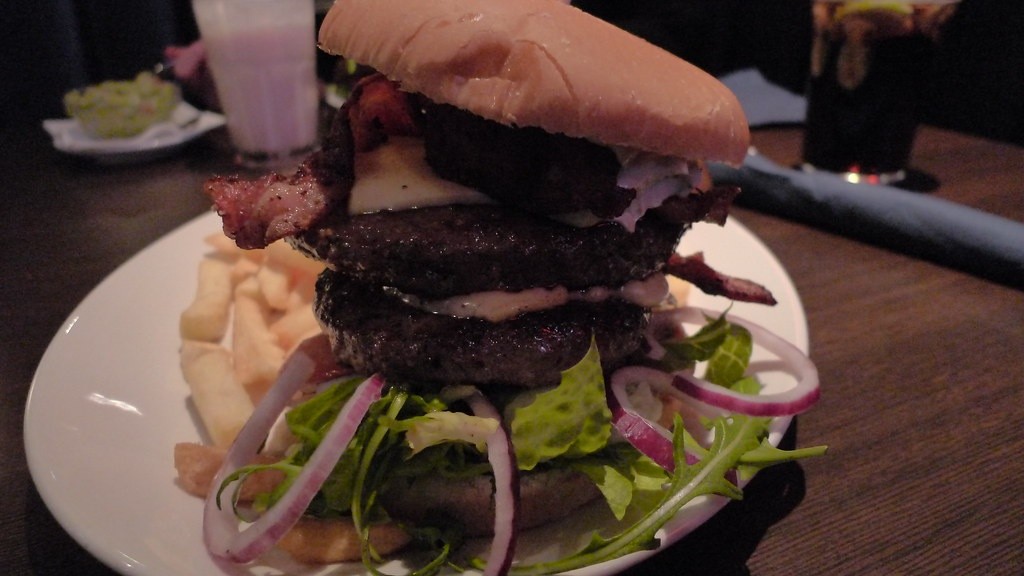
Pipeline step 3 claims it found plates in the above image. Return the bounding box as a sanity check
[23,210,809,576]
[42,99,226,154]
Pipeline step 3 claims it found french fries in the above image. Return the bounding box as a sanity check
[172,229,412,563]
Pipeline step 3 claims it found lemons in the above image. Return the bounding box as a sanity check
[831,0,915,28]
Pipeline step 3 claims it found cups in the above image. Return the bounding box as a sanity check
[192,0,321,170]
[799,0,958,187]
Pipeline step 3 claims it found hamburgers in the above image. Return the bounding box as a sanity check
[204,0,823,576]
